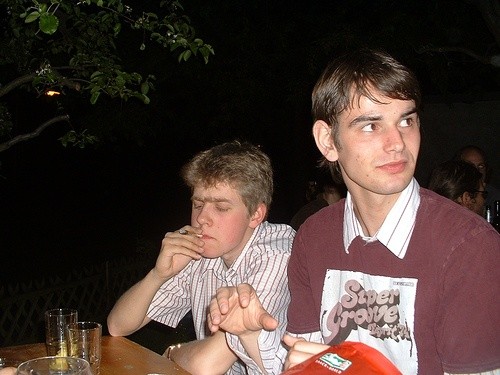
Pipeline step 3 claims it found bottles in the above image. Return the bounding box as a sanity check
[485,206,491,225]
[493,201,500,233]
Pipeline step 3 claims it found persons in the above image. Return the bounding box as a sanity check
[458,144,487,181]
[280,48,500,375]
[287,147,348,232]
[425,153,488,220]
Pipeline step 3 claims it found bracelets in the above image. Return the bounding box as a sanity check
[106,140,297,375]
[167,344,186,361]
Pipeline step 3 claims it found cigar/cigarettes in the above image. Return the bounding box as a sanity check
[178,228,204,239]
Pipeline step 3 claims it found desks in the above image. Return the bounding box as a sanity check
[0,334,192,375]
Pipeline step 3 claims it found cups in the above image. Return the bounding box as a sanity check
[66,321,102,375]
[44,308,78,357]
[17,356,92,375]
